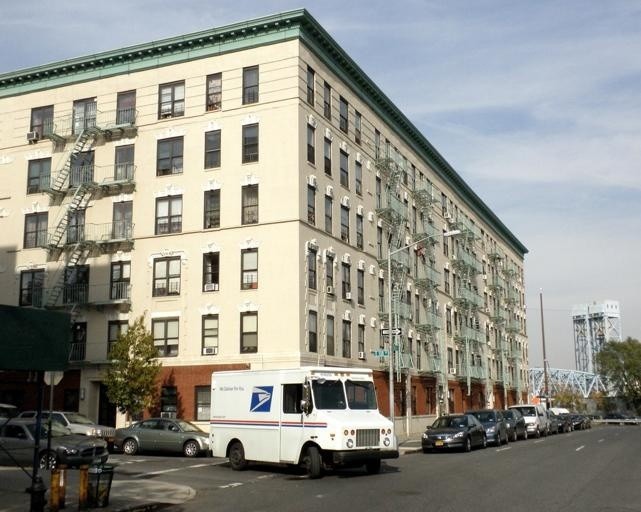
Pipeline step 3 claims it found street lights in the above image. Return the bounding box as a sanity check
[387,229,463,437]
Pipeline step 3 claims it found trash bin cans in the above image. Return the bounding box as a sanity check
[89,465,114,508]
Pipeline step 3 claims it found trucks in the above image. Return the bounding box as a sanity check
[210,366,399,480]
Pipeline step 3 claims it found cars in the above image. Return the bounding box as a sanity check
[603,413,636,425]
[421,413,488,453]
[463,403,592,447]
[114,418,211,457]
[0,404,116,469]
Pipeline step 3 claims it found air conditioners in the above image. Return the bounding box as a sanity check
[326,284,335,296]
[27,131,39,144]
[358,350,367,360]
